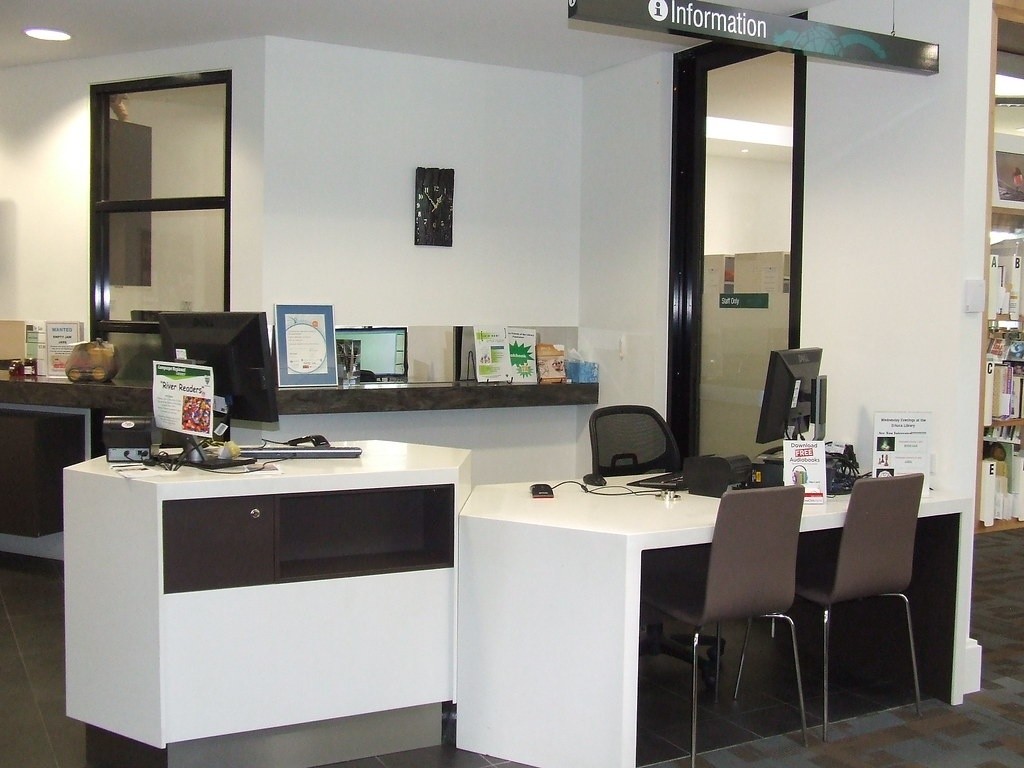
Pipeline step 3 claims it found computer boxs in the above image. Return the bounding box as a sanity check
[750,457,843,491]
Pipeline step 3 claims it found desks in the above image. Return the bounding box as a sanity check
[457,467,977,768]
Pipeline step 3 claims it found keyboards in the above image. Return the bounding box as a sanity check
[239,447,363,460]
[640,473,684,486]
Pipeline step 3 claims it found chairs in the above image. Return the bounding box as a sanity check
[587,402,725,690]
[732,470,924,741]
[640,483,808,768]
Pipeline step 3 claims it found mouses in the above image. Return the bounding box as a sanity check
[583,474,607,486]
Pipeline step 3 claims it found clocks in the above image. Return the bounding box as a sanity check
[414,167,454,247]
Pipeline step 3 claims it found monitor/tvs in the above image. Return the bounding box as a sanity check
[755,347,827,459]
[98,320,162,380]
[153,310,279,470]
[335,327,408,383]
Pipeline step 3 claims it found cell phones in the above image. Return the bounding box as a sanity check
[531,484,554,498]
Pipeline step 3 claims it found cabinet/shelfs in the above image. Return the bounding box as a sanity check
[973,0,1024,535]
